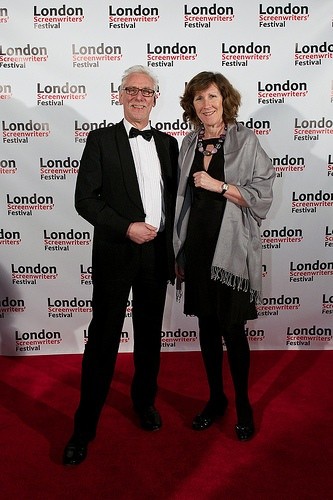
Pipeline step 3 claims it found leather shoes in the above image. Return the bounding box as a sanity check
[133,399,162,432]
[235,407,254,442]
[63,429,96,465]
[192,395,228,431]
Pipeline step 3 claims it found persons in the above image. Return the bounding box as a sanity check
[171,71,277,441]
[62,65,180,465]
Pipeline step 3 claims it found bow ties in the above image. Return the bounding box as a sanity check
[129,127,154,142]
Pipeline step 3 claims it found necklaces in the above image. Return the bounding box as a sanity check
[196,124,230,156]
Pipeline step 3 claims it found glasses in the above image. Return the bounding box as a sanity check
[121,87,155,97]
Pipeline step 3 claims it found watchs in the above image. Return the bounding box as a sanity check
[219,181,229,195]
